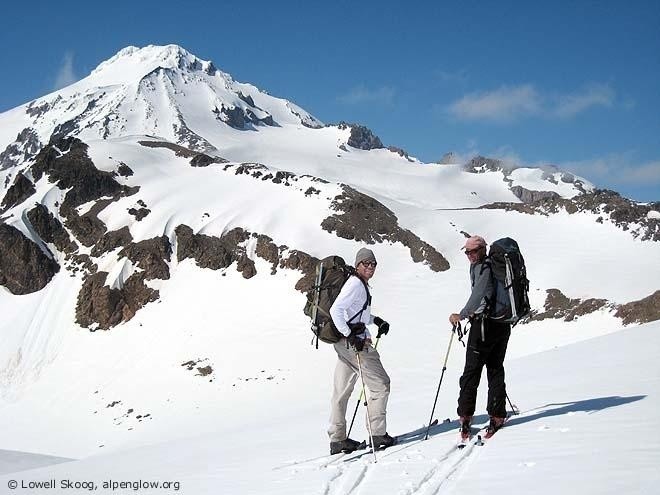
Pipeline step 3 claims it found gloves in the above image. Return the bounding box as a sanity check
[374,316,390,335]
[346,332,364,352]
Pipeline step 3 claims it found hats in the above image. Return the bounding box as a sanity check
[355,247,376,267]
[460,235,488,252]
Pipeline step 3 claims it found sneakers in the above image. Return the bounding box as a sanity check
[330,438,361,454]
[368,431,398,448]
[488,417,497,434]
[461,419,472,434]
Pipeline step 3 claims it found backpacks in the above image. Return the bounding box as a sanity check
[304,255,372,349]
[472,237,531,328]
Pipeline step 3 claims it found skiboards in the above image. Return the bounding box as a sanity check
[270,405,518,472]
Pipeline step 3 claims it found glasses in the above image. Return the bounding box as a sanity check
[361,260,377,267]
[465,247,484,255]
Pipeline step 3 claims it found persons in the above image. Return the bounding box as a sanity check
[450,235,512,439]
[328,247,394,456]
